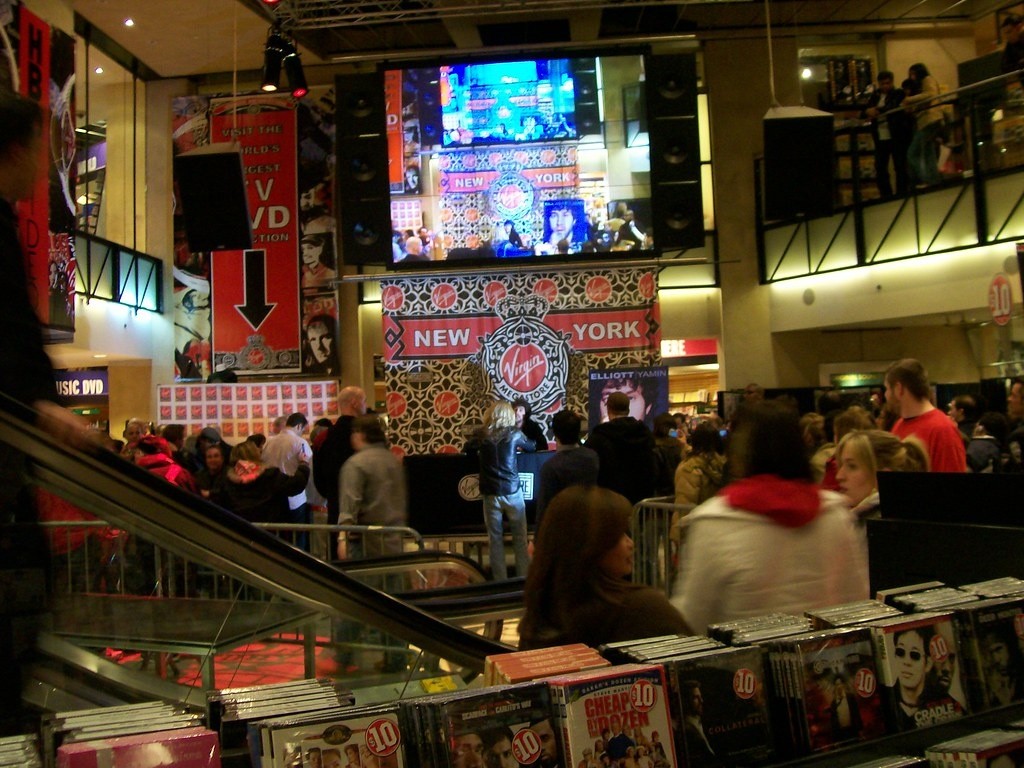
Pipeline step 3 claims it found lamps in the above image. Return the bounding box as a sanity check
[261,24,308,98]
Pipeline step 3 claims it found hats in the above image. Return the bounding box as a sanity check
[127,437,157,454]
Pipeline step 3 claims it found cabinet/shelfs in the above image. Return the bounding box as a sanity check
[75,129,106,177]
[836,98,1024,181]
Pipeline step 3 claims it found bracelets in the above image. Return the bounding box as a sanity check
[337,536,346,542]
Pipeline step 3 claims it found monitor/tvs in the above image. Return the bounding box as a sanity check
[386,42,656,272]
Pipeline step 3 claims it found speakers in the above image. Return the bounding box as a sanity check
[334,71,390,268]
[173,140,253,254]
[650,51,705,254]
[762,105,835,218]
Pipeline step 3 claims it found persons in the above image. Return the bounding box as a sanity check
[0,95,84,730]
[389,80,654,266]
[518,356,1024,660]
[683,622,1024,768]
[37,386,410,671]
[862,64,947,197]
[460,398,535,578]
[593,367,658,427]
[512,398,549,451]
[280,715,673,768]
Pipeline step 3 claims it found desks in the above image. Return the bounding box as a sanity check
[47,591,329,689]
[402,450,557,535]
